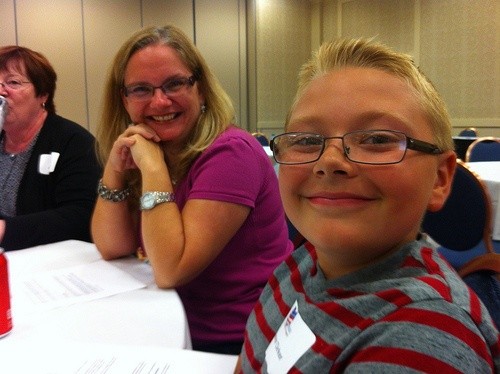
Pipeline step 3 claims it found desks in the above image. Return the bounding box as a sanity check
[456,159,500,244]
[247,129,500,321]
[263,147,280,179]
[451,135,476,160]
[0,240,191,349]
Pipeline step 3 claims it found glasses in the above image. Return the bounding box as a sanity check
[267,128,445,165]
[121,73,196,102]
[1,77,33,91]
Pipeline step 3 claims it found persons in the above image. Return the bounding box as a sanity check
[0,45,105,253]
[92,24,295,356]
[232,34,500,374]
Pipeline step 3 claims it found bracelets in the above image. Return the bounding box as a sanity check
[98,178,130,202]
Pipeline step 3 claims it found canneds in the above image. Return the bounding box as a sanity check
[0,247,12,340]
[0,96,10,136]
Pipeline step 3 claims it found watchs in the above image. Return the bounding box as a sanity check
[140,192,176,210]
